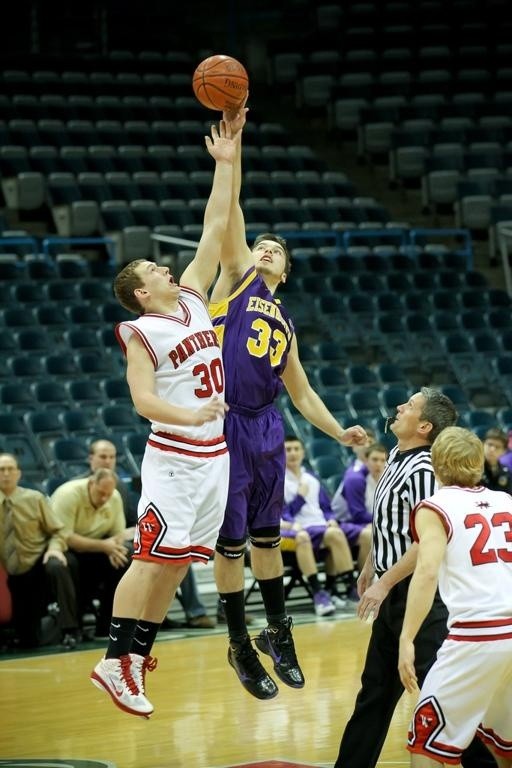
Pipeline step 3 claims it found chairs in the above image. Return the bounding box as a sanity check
[1,0,511,647]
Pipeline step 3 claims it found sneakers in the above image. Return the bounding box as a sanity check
[255,624,304,688]
[91,653,154,719]
[228,640,278,699]
[314,590,335,616]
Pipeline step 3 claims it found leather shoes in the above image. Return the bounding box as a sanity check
[189,615,214,628]
[217,614,254,625]
[93,624,111,640]
[63,631,82,650]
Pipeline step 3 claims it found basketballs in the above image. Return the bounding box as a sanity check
[193,55,249,111]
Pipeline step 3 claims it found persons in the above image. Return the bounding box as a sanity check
[282,435,371,615]
[331,383,502,768]
[1,452,80,656]
[49,466,133,642]
[354,433,376,466]
[397,421,512,768]
[477,427,511,494]
[203,88,369,701]
[332,445,387,580]
[135,462,255,630]
[279,499,335,615]
[89,119,246,721]
[72,439,181,632]
